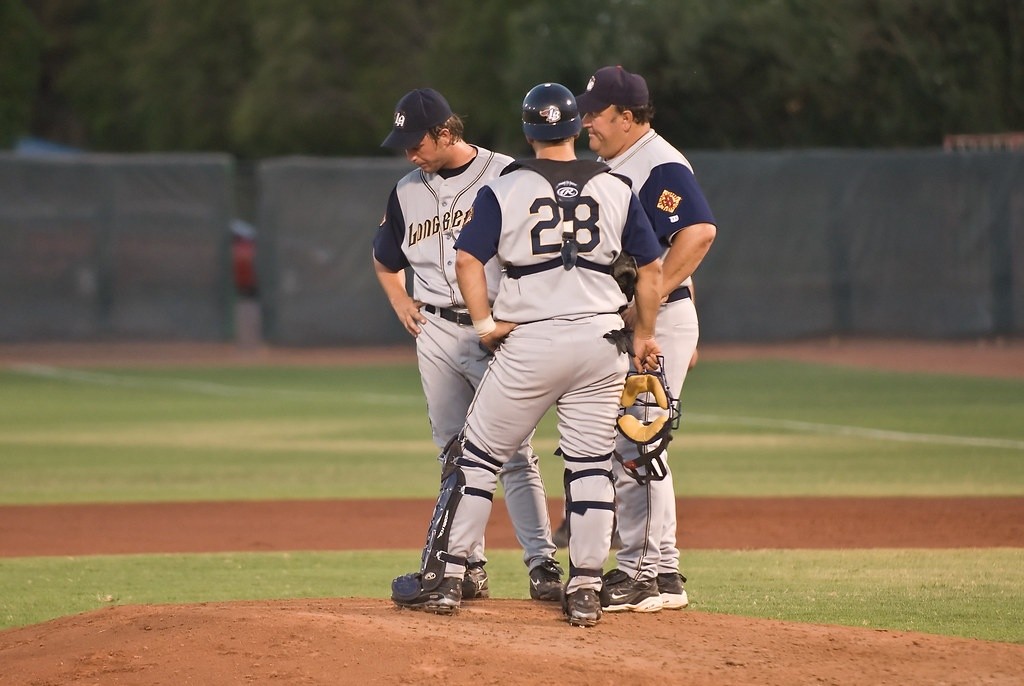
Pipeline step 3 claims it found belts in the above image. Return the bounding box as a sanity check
[663,286,692,304]
[424,304,474,326]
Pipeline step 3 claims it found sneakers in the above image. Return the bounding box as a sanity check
[601,568,689,613]
[529,560,566,601]
[566,588,602,627]
[390,576,463,615]
[463,565,490,600]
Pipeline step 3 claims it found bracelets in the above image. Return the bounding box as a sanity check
[472,314,496,337]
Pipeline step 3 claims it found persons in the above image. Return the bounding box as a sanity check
[392,83,662,626]
[575,65,717,612]
[373,87,565,601]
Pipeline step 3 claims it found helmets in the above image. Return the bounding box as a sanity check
[523,81,584,142]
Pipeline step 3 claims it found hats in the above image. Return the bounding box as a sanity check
[380,88,454,152]
[574,63,650,113]
